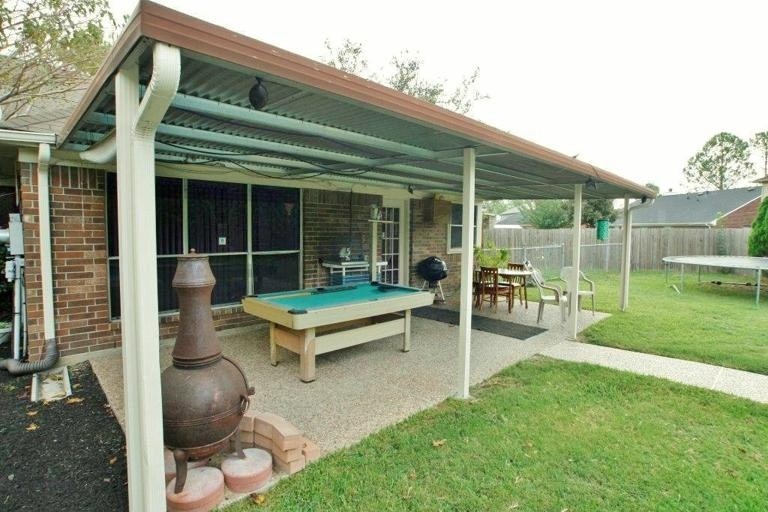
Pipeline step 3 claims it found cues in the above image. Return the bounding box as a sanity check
[259,285,358,300]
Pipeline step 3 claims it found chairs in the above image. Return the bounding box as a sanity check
[471,259,596,325]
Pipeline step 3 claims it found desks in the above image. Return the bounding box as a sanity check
[243,260,436,382]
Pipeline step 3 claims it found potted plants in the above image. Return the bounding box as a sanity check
[580,198,619,241]
